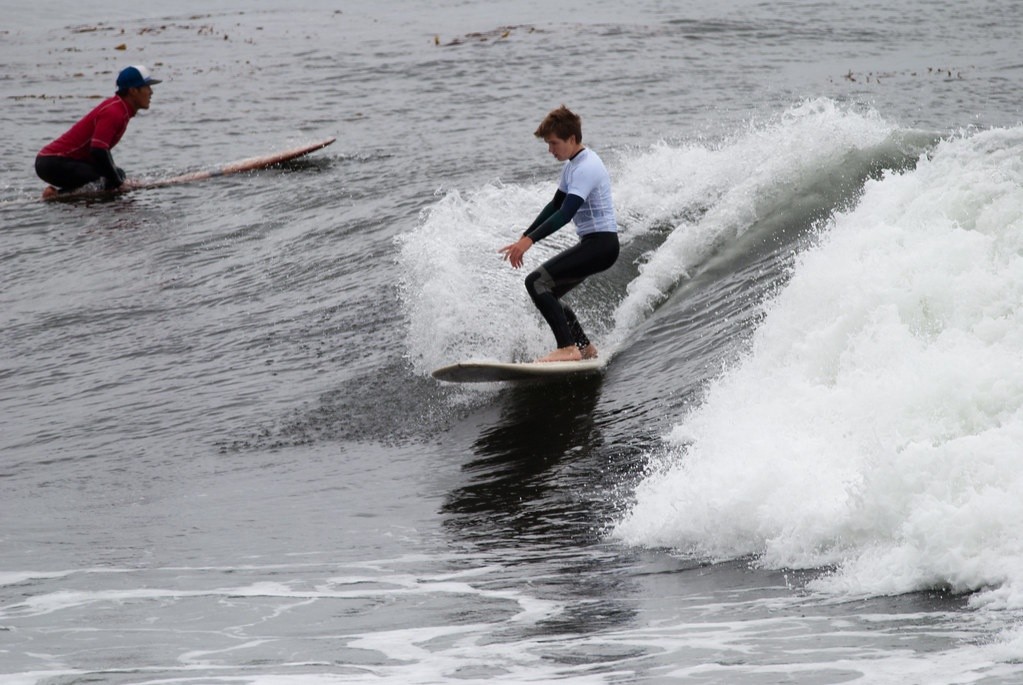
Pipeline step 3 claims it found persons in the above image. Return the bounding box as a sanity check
[35,65,162,197]
[499,105,620,362]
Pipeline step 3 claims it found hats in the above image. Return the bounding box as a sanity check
[117,66,163,87]
